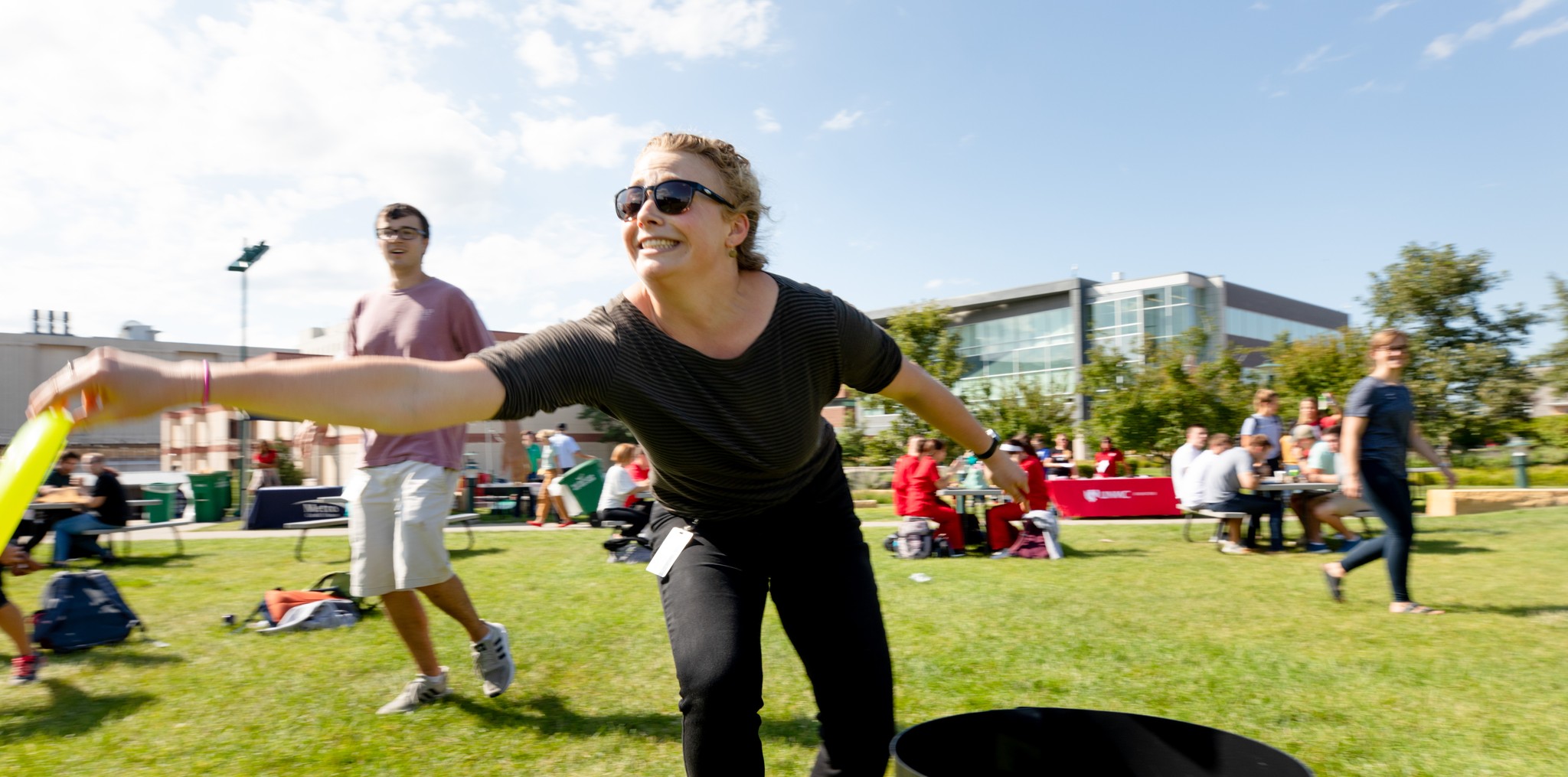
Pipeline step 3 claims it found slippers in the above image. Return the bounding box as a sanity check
[1323,566,1342,603]
[1388,600,1441,615]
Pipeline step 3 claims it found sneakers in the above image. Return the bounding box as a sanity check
[374,665,450,717]
[469,619,513,697]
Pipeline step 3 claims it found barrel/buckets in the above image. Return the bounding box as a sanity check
[887,706,1314,777]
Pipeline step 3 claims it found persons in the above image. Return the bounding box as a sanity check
[29,131,1029,777]
[0,546,38,686]
[1202,434,1295,550]
[1240,388,1295,538]
[1170,424,1231,542]
[45,452,129,568]
[1286,392,1374,552]
[526,428,575,529]
[0,442,46,555]
[986,429,1046,558]
[291,202,533,716]
[1320,332,1459,617]
[1043,434,1079,479]
[548,422,598,474]
[46,452,83,529]
[247,438,282,493]
[598,442,653,550]
[1094,436,1132,478]
[520,430,542,517]
[892,434,967,557]
[1030,432,1048,474]
[1181,433,1245,555]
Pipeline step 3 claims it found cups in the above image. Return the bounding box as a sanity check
[1273,471,1286,484]
[1288,470,1300,477]
[936,465,948,479]
[956,472,968,482]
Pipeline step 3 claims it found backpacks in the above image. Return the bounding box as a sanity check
[1007,510,1064,561]
[305,571,378,614]
[29,569,147,656]
[894,514,940,560]
[230,586,375,638]
[958,513,980,545]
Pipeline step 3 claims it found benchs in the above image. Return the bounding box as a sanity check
[283,517,350,559]
[1349,511,1380,538]
[446,512,484,534]
[81,518,197,567]
[1175,504,1246,545]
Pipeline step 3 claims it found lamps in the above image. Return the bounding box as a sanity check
[1504,435,1534,456]
[463,457,482,480]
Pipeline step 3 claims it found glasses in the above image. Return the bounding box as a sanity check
[1061,438,1069,442]
[1375,343,1410,352]
[376,227,427,240]
[614,179,737,222]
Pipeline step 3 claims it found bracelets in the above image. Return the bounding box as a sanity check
[201,358,211,403]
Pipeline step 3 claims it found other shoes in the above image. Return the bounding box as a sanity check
[988,548,1019,559]
[525,520,542,527]
[1334,533,1363,552]
[1217,539,1250,555]
[558,521,574,527]
[1303,543,1331,553]
[11,647,44,687]
[937,549,967,557]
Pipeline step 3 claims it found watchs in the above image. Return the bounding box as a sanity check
[973,427,1002,460]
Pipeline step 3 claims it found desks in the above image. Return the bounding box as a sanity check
[1406,467,1443,504]
[934,488,1005,513]
[28,500,163,561]
[244,483,344,525]
[479,480,543,521]
[1255,481,1340,552]
